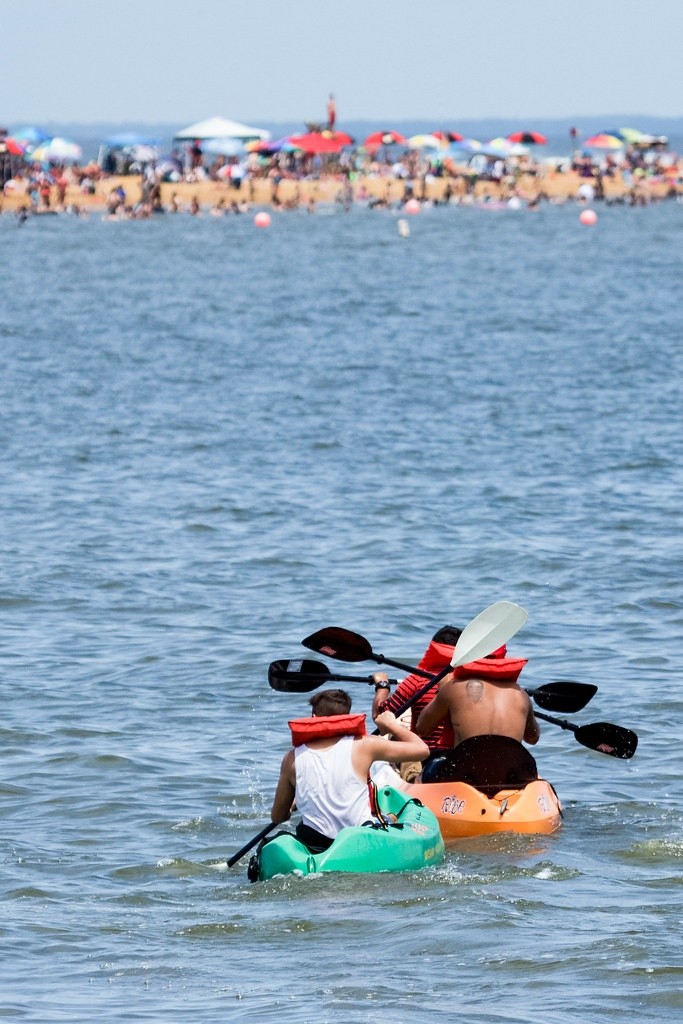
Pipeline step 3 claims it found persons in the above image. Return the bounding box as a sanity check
[372,625,463,749]
[328,94,335,130]
[415,643,540,780]
[0,135,683,219]
[271,689,430,850]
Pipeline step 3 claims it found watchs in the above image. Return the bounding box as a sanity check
[375,681,390,692]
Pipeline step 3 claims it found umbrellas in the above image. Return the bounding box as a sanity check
[13,117,648,162]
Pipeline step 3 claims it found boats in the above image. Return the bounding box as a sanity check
[256,784,445,881]
[399,777,561,839]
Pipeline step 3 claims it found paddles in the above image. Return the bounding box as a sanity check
[209,598,530,873]
[299,624,639,760]
[266,657,599,715]
